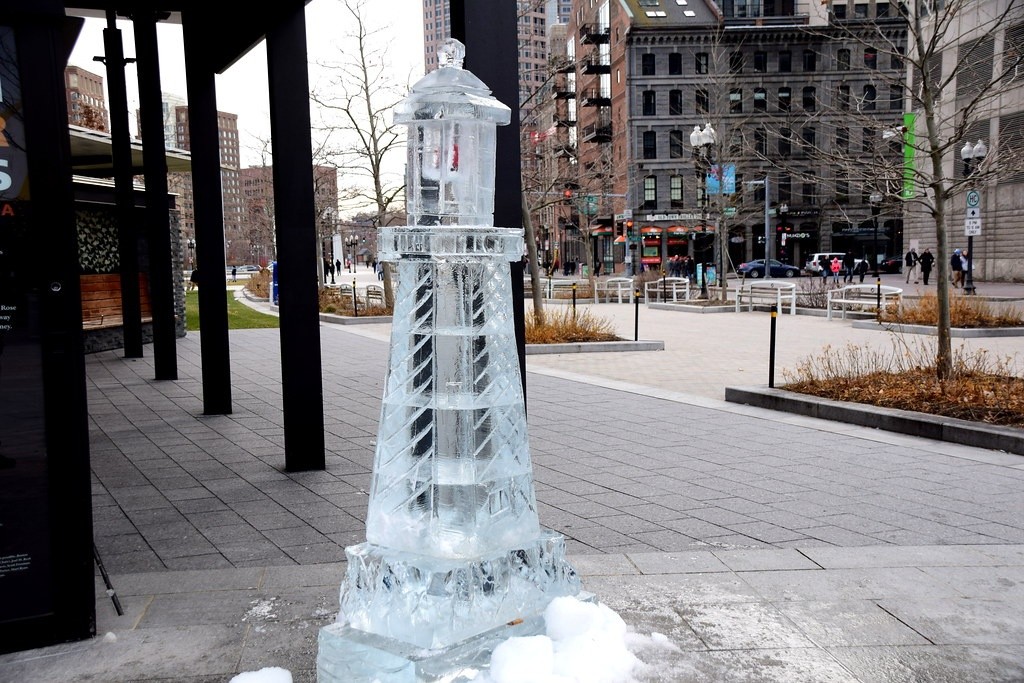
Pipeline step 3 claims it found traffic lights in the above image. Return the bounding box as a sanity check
[363,236,365,243]
[626,220,635,237]
[617,221,625,235]
[563,188,573,205]
[776,225,784,231]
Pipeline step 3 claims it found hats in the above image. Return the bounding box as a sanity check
[954,249,960,254]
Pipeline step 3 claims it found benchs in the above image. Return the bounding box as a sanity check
[524,278,549,299]
[356,284,383,307]
[594,277,633,304]
[827,283,903,322]
[736,280,796,316]
[324,284,353,304]
[81,272,178,355]
[550,279,590,299]
[645,277,690,306]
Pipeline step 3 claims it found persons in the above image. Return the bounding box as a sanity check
[324,257,384,282]
[522,254,602,279]
[190,268,198,291]
[819,247,969,287]
[664,254,694,284]
[232,266,237,283]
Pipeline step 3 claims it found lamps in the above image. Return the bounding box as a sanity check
[664,211,669,216]
[677,210,681,215]
[650,211,655,216]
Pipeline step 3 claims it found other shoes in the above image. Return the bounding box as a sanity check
[952,283,955,286]
[906,281,909,284]
[914,281,919,284]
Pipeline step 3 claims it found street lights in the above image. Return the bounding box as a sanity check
[344,236,353,273]
[188,234,195,270]
[349,235,359,273]
[780,203,789,265]
[688,121,718,300]
[868,192,883,277]
[961,139,988,297]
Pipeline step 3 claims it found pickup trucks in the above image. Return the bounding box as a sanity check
[804,252,870,277]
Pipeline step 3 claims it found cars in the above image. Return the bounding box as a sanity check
[239,265,258,272]
[736,258,802,279]
